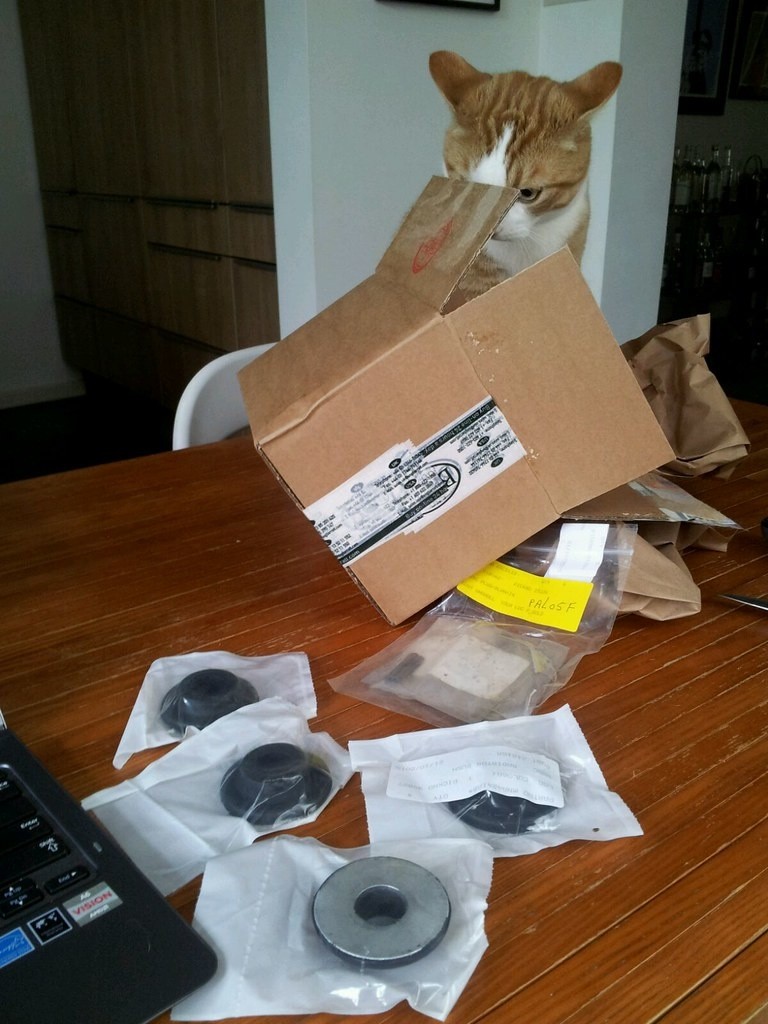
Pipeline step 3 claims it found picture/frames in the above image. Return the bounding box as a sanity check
[728,0,768,102]
[676,0,742,117]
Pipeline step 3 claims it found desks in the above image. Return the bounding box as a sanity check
[0,395,767,1024]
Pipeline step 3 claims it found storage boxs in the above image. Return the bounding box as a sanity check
[234,173,746,631]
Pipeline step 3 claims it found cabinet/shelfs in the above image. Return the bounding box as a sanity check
[12,0,280,407]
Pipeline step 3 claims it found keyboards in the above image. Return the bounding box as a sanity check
[0,726,218,1024]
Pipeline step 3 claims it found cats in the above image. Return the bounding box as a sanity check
[427,50,624,302]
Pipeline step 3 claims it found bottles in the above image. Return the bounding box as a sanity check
[659,143,734,299]
